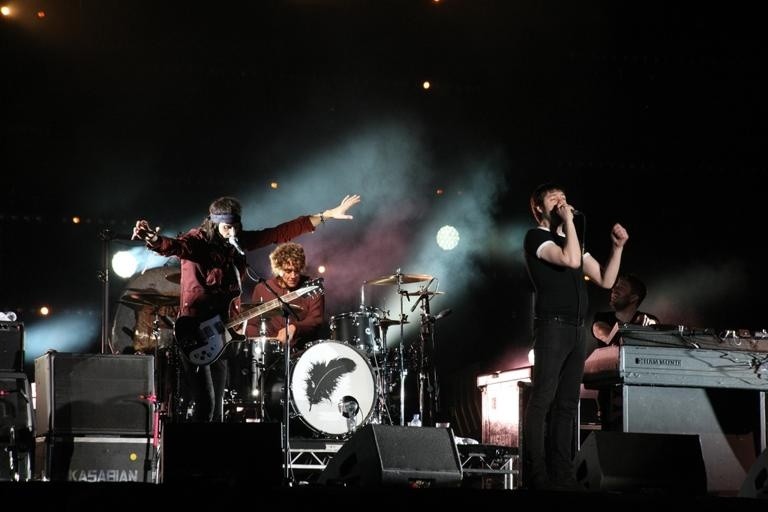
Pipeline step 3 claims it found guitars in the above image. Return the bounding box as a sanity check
[174,278,326,366]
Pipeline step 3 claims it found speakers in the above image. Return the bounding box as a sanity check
[315,422,463,488]
[0,372,36,482]
[734,446,768,498]
[0,320,25,372]
[161,421,284,488]
[43,436,159,484]
[574,430,707,496]
[34,350,157,437]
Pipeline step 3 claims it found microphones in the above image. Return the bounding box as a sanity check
[410,278,434,311]
[229,235,246,256]
[571,209,579,215]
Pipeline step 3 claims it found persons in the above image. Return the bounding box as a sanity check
[522,183,629,486]
[131,196,361,421]
[247,243,326,351]
[591,272,661,348]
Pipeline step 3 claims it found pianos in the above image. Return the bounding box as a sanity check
[583,322,767,391]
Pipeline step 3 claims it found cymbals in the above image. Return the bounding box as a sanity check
[404,290,443,296]
[379,318,409,325]
[117,287,178,308]
[367,274,432,285]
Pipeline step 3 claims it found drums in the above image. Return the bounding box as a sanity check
[228,337,285,405]
[171,373,246,424]
[329,311,386,357]
[264,340,378,441]
[155,328,192,378]
